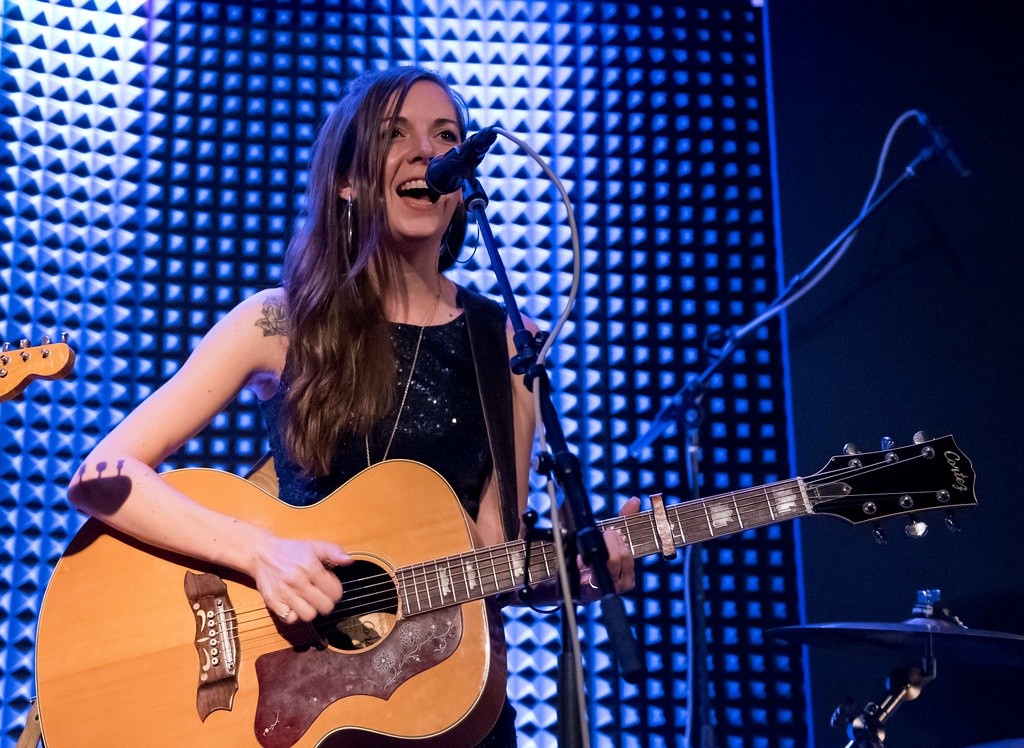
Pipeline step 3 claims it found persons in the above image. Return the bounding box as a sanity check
[68,64,641,748]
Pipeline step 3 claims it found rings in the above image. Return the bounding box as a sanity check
[279,608,290,623]
[588,574,600,590]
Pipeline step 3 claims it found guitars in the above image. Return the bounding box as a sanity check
[0,331,77,403]
[32,427,985,748]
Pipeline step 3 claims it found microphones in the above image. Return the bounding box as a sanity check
[425,126,499,195]
[917,114,975,177]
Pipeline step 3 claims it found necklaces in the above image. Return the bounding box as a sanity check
[366,271,445,467]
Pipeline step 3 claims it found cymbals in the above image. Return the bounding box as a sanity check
[762,616,1024,664]
